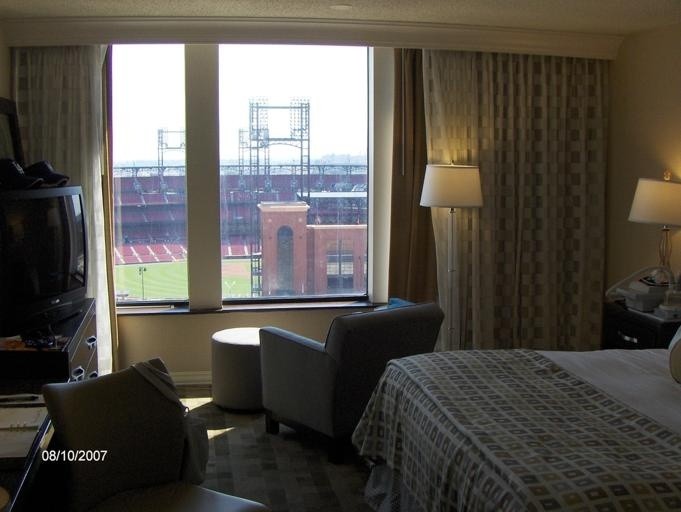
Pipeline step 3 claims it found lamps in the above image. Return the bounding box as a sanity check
[627,169,681,293]
[419,160,484,350]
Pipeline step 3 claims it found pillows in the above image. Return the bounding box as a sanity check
[668,325,681,384]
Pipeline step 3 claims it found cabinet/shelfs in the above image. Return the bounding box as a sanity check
[0,297,99,393]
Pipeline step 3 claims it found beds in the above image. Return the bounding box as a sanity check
[350,346,680,512]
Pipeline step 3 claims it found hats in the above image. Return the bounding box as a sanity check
[28,160,69,186]
[0,158,44,188]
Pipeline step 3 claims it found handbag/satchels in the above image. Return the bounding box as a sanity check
[132,361,209,486]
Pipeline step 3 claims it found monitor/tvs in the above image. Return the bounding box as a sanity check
[0,186,89,337]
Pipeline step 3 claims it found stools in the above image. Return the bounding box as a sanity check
[210,327,262,410]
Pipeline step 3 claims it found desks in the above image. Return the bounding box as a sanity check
[0,383,58,511]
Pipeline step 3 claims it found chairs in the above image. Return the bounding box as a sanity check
[42,355,271,511]
[259,299,445,466]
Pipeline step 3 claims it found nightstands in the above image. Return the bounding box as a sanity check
[601,299,681,351]
